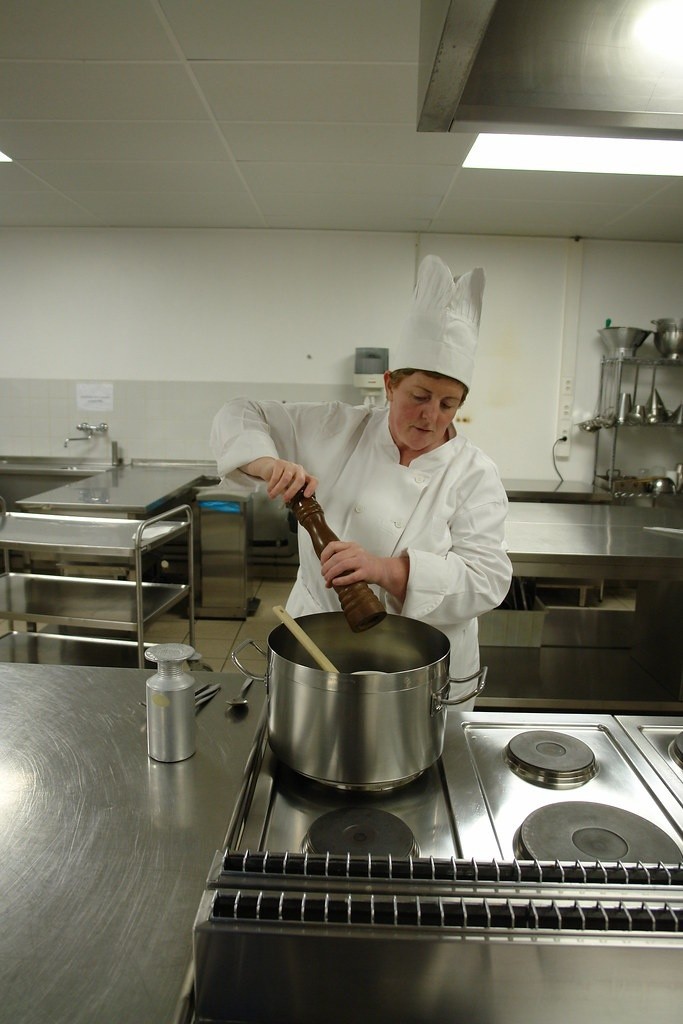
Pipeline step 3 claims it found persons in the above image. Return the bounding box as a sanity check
[210,252,514,714]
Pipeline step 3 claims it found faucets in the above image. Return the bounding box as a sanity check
[64,423,108,448]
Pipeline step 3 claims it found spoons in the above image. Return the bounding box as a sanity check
[226,679,254,705]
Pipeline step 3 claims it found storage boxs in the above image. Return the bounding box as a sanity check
[478,596,549,649]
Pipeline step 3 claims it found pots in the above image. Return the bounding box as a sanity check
[230,612,488,790]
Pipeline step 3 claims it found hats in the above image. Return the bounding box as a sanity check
[388,254,486,395]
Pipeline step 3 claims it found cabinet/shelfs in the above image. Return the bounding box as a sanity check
[593,353,683,493]
[0,502,196,669]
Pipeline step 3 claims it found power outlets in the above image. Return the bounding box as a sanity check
[559,427,570,445]
[560,403,572,420]
[562,377,575,396]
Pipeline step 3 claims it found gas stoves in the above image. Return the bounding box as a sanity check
[194,712,683,901]
